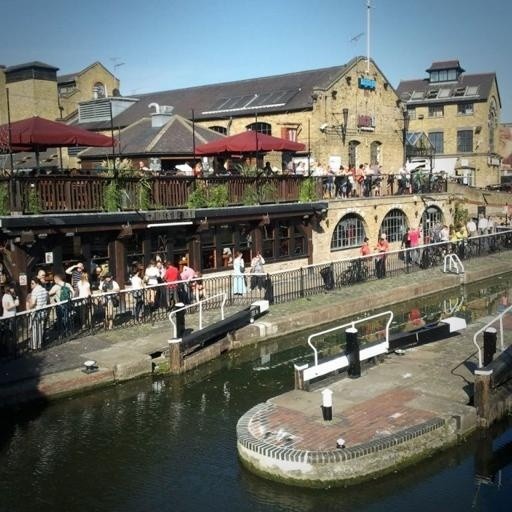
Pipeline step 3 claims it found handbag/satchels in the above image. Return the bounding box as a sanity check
[250,267,255,273]
[398,251,405,261]
[239,266,245,273]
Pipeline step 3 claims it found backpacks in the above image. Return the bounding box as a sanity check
[56,282,70,302]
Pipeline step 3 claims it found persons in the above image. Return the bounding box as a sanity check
[233,252,244,295]
[2,259,201,354]
[195,161,204,179]
[21,166,90,176]
[264,162,276,175]
[252,249,265,273]
[359,215,493,279]
[295,161,446,198]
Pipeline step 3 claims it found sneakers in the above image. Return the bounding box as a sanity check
[234,293,243,296]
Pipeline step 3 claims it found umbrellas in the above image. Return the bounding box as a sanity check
[194,130,306,155]
[0,115,117,166]
